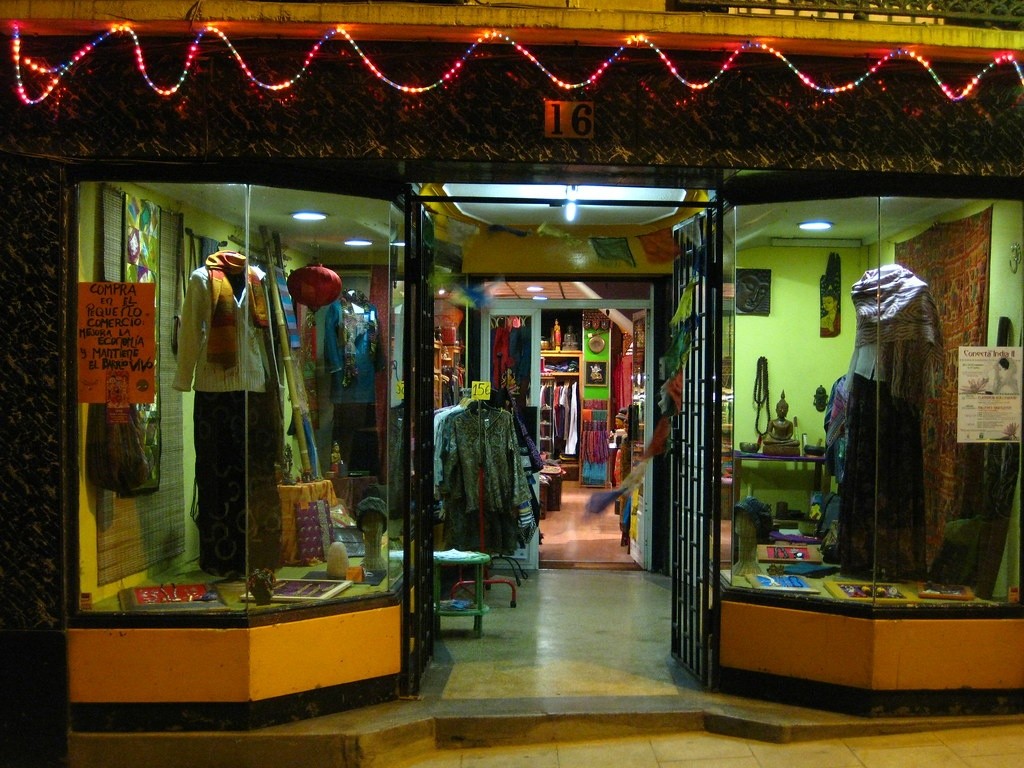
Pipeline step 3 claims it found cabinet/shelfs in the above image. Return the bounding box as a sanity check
[541,377,555,452]
[389,550,490,638]
[540,350,583,481]
[390,337,461,409]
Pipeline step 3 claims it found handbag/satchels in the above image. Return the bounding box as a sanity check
[297,501,335,564]
[88,398,153,493]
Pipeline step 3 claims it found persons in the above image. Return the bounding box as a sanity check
[763,390,803,447]
[325,290,386,477]
[550,318,581,352]
[835,263,936,578]
[172,249,275,579]
[614,409,626,433]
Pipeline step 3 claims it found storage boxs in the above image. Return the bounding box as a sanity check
[295,499,335,561]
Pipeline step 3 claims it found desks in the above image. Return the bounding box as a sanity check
[329,476,377,509]
[277,480,338,566]
[734,451,826,503]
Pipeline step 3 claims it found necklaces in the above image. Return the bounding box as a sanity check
[752,359,772,439]
[142,583,212,603]
[271,578,335,598]
[756,547,805,588]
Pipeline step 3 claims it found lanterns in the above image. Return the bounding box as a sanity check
[288,262,341,359]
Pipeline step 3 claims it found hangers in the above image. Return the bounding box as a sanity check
[458,388,501,413]
[541,380,552,389]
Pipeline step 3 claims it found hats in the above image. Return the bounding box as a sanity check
[737,497,773,538]
[356,498,387,533]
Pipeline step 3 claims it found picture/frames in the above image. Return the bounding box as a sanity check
[585,361,609,387]
